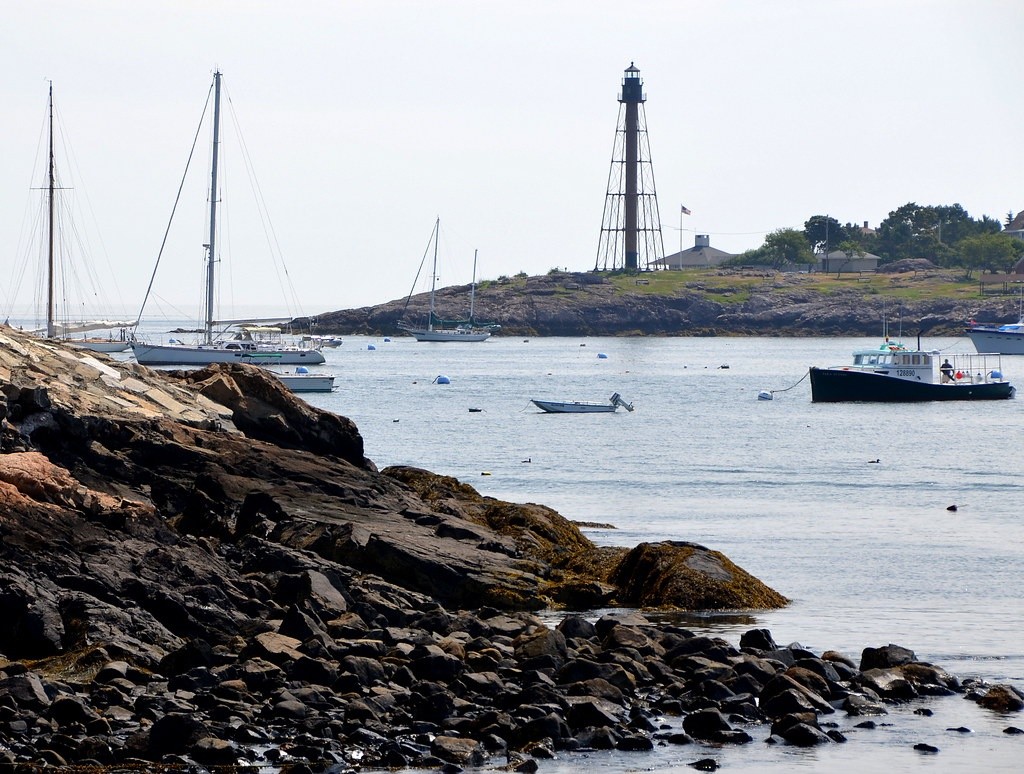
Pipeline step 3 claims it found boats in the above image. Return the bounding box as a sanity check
[312,336,342,348]
[809,300,1016,403]
[238,353,340,392]
[530,392,634,414]
[243,327,282,345]
[966,315,1024,355]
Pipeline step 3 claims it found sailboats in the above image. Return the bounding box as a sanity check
[3,79,139,351]
[397,215,491,341]
[125,63,327,364]
[433,249,503,334]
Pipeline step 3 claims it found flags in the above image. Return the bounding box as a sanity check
[681,205,691,216]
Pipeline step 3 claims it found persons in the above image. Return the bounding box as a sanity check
[940,358,955,382]
